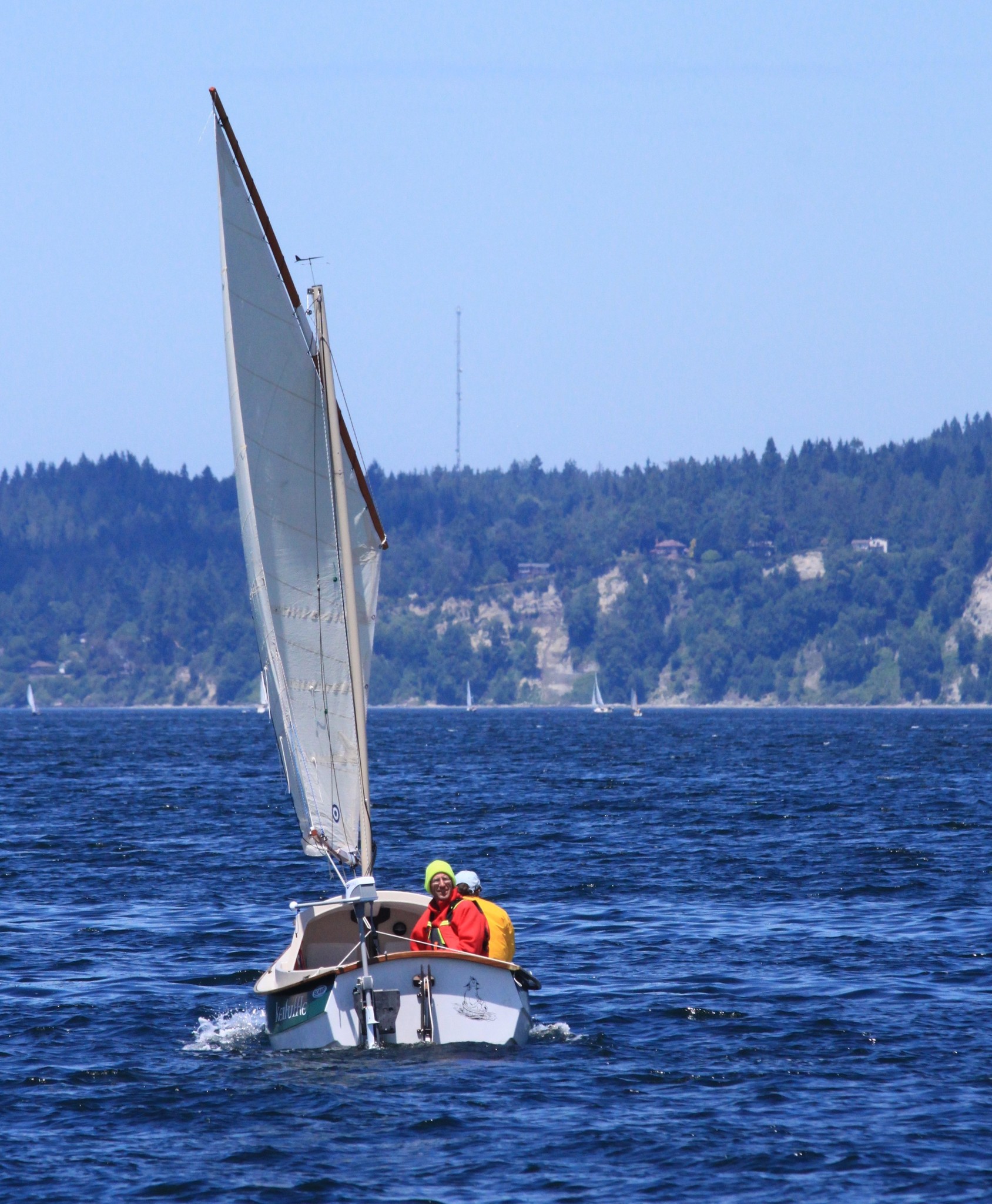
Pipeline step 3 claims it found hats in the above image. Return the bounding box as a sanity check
[454,870,483,893]
[424,860,457,894]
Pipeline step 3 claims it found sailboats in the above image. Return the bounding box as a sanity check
[591,673,613,712]
[207,81,548,1054]
[630,689,644,717]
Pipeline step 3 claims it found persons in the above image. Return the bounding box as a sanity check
[455,870,516,963]
[410,860,489,957]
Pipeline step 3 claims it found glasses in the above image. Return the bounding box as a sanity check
[431,875,451,886]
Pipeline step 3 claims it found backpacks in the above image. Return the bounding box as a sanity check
[432,895,515,963]
